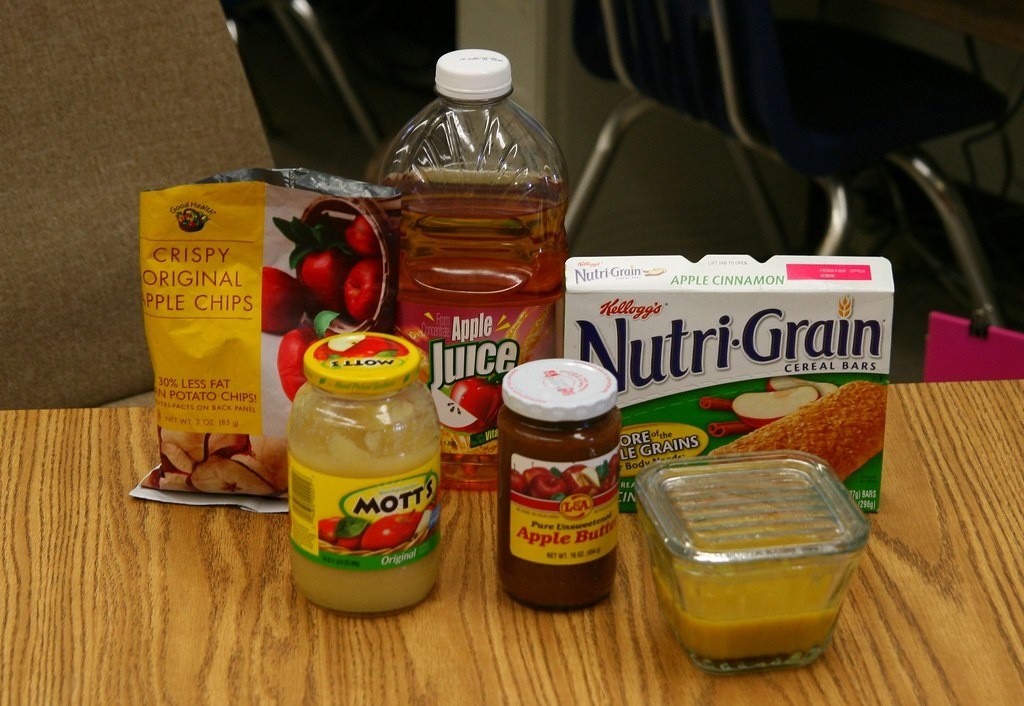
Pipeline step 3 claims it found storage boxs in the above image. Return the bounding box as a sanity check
[635,450,869,671]
[586,255,894,490]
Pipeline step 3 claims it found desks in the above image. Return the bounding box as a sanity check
[0,379,1024,706]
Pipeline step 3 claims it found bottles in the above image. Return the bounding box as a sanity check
[379,50,569,492]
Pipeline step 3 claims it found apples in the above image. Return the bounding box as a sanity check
[343,260,381,318]
[511,464,527,494]
[299,250,346,314]
[524,461,547,486]
[361,508,422,550]
[319,518,362,550]
[261,267,302,335]
[346,216,380,255]
[564,465,590,496]
[531,473,566,499]
[277,328,338,401]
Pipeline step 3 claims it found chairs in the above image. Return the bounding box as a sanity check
[562,0,1012,327]
[219,0,379,182]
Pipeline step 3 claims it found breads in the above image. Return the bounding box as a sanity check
[707,377,886,483]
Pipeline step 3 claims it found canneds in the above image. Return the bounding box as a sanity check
[497,359,621,609]
[287,334,442,613]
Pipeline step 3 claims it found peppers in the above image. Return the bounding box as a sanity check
[450,375,504,427]
